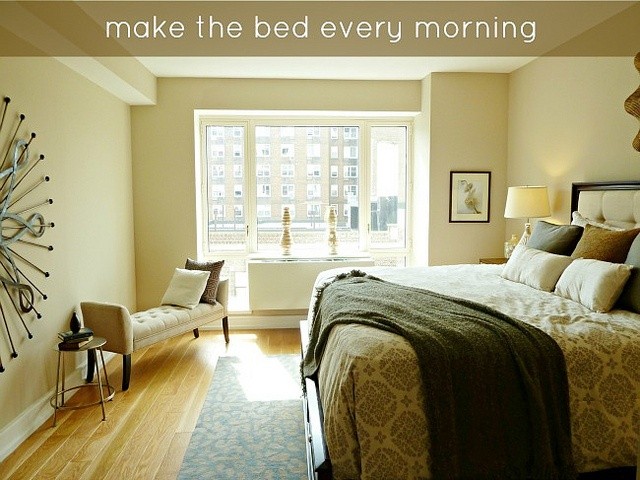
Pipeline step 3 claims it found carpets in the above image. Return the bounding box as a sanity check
[175,353,310,480]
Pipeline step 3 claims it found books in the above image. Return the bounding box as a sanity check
[58,341,90,351]
[68,335,93,341]
[57,327,94,341]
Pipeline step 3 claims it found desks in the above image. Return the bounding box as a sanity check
[246,247,374,316]
[49,337,116,427]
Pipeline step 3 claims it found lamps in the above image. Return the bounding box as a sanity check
[503,185,551,246]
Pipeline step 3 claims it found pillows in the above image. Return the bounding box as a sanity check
[527,220,584,257]
[184,258,225,306]
[570,223,640,263]
[556,257,633,313]
[160,267,210,309]
[570,210,625,232]
[500,245,573,292]
[624,233,640,314]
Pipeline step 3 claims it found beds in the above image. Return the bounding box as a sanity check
[300,181,639,480]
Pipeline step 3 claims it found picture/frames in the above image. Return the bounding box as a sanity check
[449,171,491,225]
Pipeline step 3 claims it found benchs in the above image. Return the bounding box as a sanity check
[78,279,230,390]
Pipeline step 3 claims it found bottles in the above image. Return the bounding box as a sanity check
[510,233,519,247]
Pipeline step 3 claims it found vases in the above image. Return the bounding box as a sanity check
[281,207,293,256]
[325,205,338,255]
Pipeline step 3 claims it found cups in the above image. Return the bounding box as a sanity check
[505,241,514,258]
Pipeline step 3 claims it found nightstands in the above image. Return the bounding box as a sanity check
[478,258,509,264]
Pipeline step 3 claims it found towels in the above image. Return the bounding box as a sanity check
[300,269,575,480]
